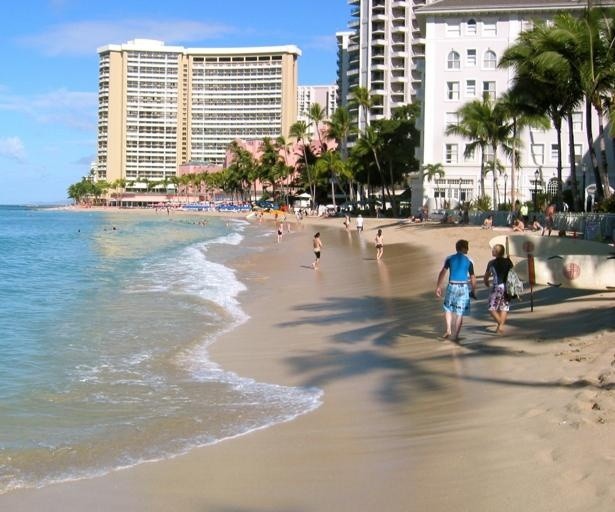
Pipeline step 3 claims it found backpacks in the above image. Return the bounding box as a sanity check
[506,269,524,298]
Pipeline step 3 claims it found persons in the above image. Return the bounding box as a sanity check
[412,207,423,222]
[312,232,323,271]
[484,244,513,334]
[80,201,91,208]
[440,210,464,224]
[479,215,493,230]
[376,229,384,264]
[507,201,569,237]
[153,200,381,245]
[435,239,478,341]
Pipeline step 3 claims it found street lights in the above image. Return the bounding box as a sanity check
[534,170,539,205]
[504,173,508,203]
[581,163,588,212]
